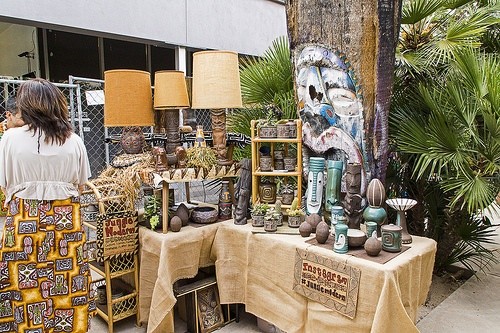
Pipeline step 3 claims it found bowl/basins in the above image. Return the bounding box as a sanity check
[348,229,365,247]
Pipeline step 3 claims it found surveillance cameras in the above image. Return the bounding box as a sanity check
[18,52,28,57]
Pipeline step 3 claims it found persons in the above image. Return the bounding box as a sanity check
[0,77,92,333]
[0,96,25,140]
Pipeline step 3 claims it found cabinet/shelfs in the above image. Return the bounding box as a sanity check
[251,118,304,215]
[79,178,142,331]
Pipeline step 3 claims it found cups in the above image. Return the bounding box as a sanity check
[381,224,402,252]
[111,291,124,309]
[97,286,107,305]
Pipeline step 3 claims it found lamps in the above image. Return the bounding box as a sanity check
[101,70,155,166]
[152,69,195,162]
[190,51,243,172]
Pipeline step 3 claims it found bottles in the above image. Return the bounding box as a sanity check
[333,216,348,253]
[218,181,232,218]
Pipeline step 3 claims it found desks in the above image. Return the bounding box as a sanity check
[110,198,438,333]
